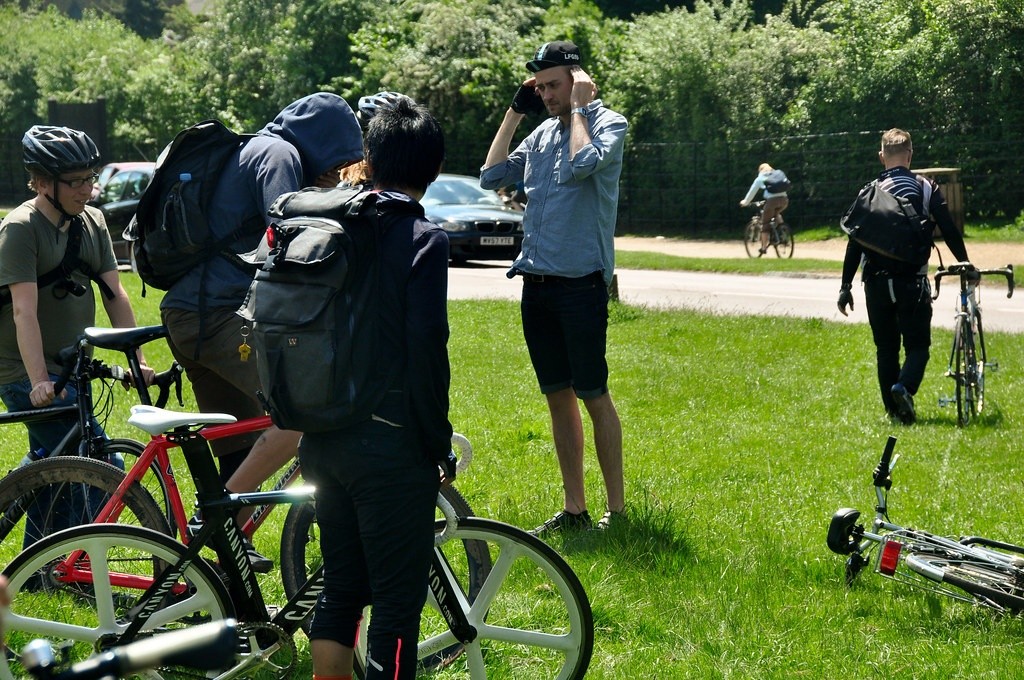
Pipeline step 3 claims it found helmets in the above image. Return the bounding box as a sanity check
[21,124,101,176]
[357,90,419,133]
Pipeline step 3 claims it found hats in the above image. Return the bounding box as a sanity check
[525,41,579,74]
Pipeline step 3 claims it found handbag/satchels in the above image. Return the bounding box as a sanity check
[762,174,792,194]
[839,173,945,272]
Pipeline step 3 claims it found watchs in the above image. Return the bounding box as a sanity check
[571,107,588,118]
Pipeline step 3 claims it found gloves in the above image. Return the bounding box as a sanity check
[964,264,981,287]
[837,288,854,316]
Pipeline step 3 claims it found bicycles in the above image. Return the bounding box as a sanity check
[0,402,595,680]
[827,435,1024,622]
[0,325,491,680]
[740,200,795,259]
[931,262,1016,428]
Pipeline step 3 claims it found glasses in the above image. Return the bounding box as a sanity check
[50,172,100,187]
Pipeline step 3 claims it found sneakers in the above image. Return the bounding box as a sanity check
[886,413,901,422]
[586,506,628,532]
[891,383,915,425]
[188,507,274,573]
[523,511,592,541]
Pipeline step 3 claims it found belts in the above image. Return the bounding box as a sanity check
[521,271,562,285]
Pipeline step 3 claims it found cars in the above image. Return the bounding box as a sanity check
[88,162,167,268]
[415,172,525,265]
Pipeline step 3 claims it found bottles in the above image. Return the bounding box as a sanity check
[9,448,44,471]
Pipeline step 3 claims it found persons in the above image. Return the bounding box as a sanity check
[298,94,451,680]
[838,128,981,426]
[160,92,364,571]
[740,163,790,254]
[480,43,627,535]
[0,127,156,591]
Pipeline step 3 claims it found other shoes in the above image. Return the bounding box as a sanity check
[759,248,766,254]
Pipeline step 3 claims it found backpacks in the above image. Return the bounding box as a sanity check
[122,116,268,295]
[234,184,417,435]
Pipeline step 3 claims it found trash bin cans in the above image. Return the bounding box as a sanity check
[910,167,965,239]
